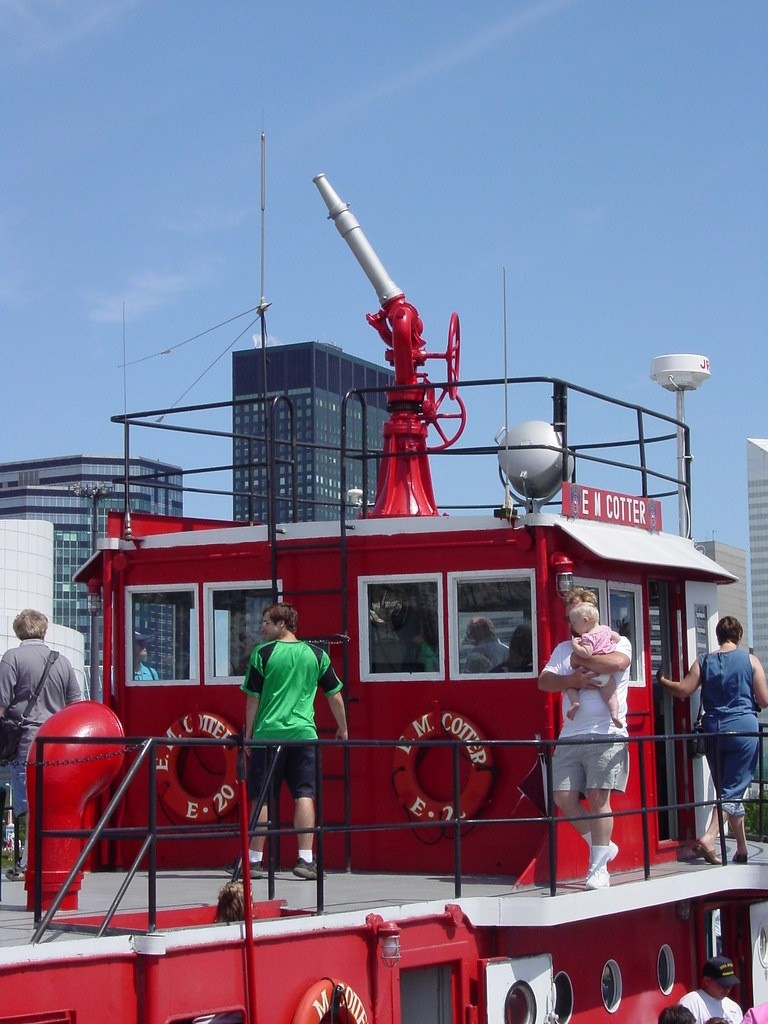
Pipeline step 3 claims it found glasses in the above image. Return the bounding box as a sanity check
[262,621,272,626]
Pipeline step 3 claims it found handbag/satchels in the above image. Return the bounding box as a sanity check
[687,722,706,758]
[0,716,21,767]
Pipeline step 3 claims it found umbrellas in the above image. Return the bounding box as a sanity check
[511,735,548,819]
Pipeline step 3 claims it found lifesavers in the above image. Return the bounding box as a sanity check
[392,707,493,827]
[152,713,246,826]
[292,975,368,1024]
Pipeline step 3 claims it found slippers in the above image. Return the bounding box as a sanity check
[733,851,747,864]
[692,839,723,865]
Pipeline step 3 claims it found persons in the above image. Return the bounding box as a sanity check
[192,880,255,1024]
[133,630,159,681]
[658,956,768,1024]
[223,602,349,879]
[461,616,532,673]
[0,609,81,881]
[390,605,440,673]
[537,587,632,890]
[6,834,13,862]
[656,616,768,865]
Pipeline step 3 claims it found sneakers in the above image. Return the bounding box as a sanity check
[5,857,26,881]
[585,840,619,890]
[224,857,262,879]
[293,853,327,880]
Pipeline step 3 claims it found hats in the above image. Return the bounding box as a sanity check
[135,631,150,641]
[703,955,741,987]
